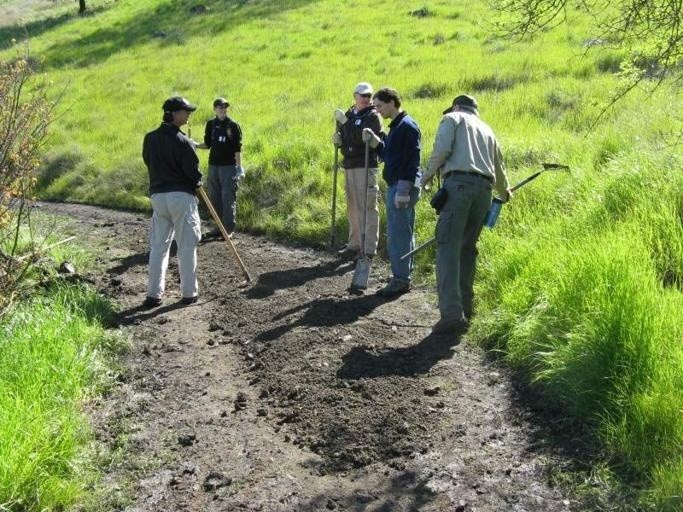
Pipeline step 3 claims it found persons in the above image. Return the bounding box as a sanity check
[333,83,383,261]
[141,96,206,305]
[189,98,245,241]
[419,94,512,332]
[361,88,422,298]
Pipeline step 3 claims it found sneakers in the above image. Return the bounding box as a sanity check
[144,296,162,307]
[432,317,468,335]
[222,230,234,240]
[182,296,197,305]
[337,246,357,257]
[381,278,412,296]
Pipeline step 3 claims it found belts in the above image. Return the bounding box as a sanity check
[444,171,493,184]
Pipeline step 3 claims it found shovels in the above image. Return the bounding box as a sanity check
[351,139,373,289]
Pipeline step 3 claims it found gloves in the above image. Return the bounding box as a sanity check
[394,180,414,209]
[334,109,348,125]
[231,165,245,182]
[330,131,343,148]
[420,167,436,193]
[362,127,381,149]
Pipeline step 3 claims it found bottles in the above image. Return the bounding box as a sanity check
[414,173,421,196]
[485,198,502,229]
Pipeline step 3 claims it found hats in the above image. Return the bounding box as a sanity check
[353,83,373,95]
[213,98,229,107]
[443,95,477,115]
[162,97,196,112]
